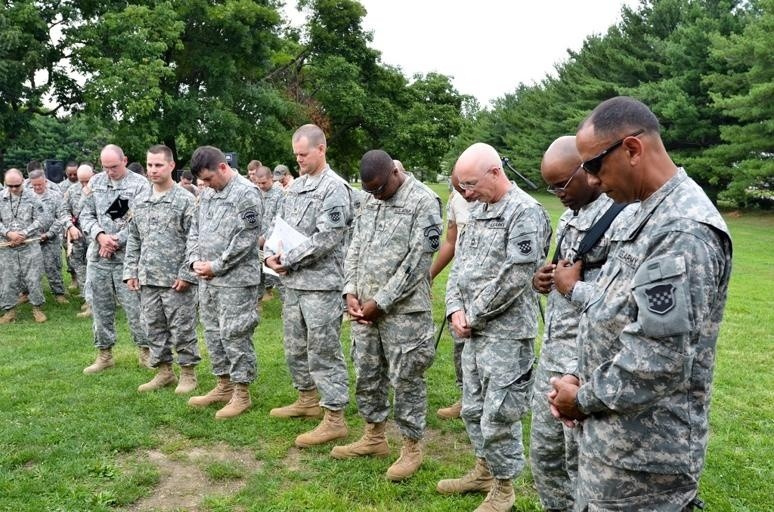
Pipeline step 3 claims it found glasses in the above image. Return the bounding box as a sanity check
[459,167,501,190]
[581,130,645,174]
[362,168,394,195]
[547,162,581,194]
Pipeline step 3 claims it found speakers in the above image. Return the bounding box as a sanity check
[44,160,63,183]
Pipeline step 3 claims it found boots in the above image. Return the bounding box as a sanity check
[295,407,348,447]
[216,383,252,418]
[0,279,94,322]
[268,391,322,417]
[385,437,423,480]
[188,374,234,408]
[435,458,515,511]
[332,420,390,459]
[84,347,114,374]
[255,287,273,312]
[176,364,198,395]
[436,400,463,418]
[138,346,178,392]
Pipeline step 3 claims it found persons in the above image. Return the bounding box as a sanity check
[128,162,146,175]
[121,144,200,396]
[177,170,209,199]
[244,160,292,303]
[189,146,263,419]
[525,132,639,511]
[393,157,408,174]
[264,123,352,451]
[330,148,444,482]
[428,163,475,419]
[0,158,91,324]
[435,144,551,511]
[546,95,733,512]
[80,143,154,375]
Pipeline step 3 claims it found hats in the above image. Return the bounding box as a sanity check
[272,165,288,181]
